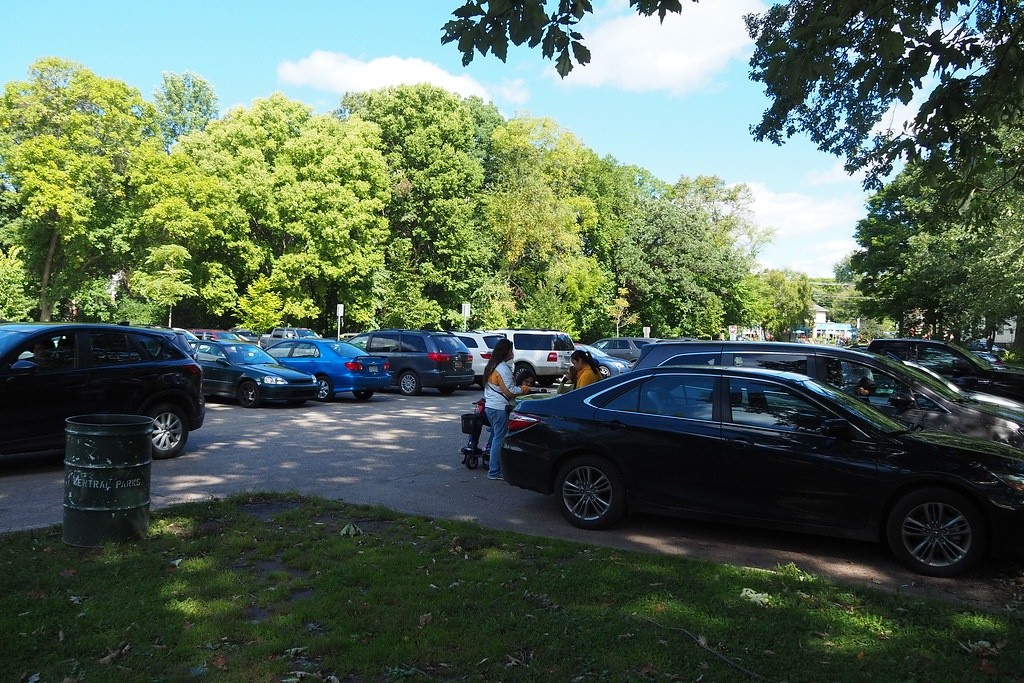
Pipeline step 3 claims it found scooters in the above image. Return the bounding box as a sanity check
[459,397,495,470]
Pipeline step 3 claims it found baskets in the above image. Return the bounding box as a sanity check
[460,413,485,434]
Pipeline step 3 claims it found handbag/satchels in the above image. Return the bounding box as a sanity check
[487,383,515,415]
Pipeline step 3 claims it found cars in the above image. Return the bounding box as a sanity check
[188,340,322,412]
[575,343,635,381]
[340,333,358,342]
[151,326,339,363]
[588,337,1024,460]
[499,364,1024,578]
[0,320,206,459]
[261,338,393,401]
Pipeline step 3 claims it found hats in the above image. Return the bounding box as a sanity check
[515,368,527,378]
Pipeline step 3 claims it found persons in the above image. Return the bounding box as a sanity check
[31,338,57,365]
[867,338,873,347]
[517,364,536,399]
[483,338,531,489]
[551,350,605,394]
[839,337,852,346]
[801,333,807,343]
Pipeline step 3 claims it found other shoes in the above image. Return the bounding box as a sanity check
[487,471,504,481]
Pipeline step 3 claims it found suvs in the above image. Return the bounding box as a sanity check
[477,328,578,387]
[436,331,508,386]
[346,328,476,399]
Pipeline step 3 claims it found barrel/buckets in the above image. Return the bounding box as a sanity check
[62,413,154,547]
